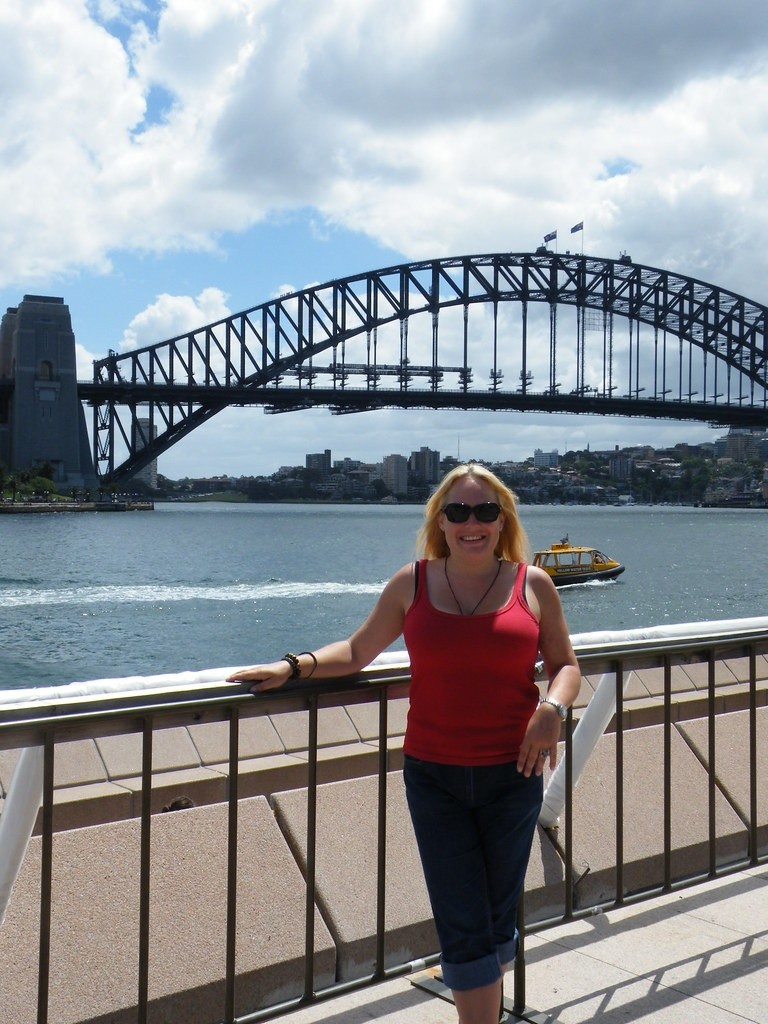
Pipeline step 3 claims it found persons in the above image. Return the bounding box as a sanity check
[595,554,604,564]
[227,463,581,1024]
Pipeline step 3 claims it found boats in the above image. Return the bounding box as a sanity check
[532,542,626,588]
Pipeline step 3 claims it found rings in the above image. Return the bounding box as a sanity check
[540,749,550,758]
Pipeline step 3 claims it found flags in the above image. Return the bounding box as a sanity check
[544,230,557,242]
[570,221,584,233]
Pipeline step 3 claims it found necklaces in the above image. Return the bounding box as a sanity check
[444,557,503,616]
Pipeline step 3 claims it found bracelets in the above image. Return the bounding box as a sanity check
[285,653,300,680]
[299,652,317,678]
[281,657,296,680]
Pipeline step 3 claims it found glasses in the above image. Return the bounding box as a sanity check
[440,503,502,523]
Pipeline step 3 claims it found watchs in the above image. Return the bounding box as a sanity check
[540,696,568,720]
